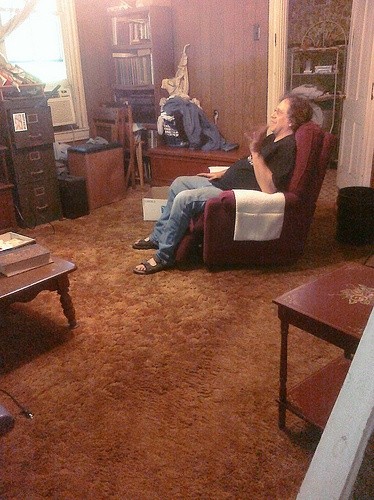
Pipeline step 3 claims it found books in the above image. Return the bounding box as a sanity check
[111,16,154,86]
[0,244,54,276]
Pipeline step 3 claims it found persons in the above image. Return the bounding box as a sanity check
[133,94,312,274]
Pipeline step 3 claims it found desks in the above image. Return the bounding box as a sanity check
[271,261,374,486]
[67,141,125,209]
[0,255,79,330]
[145,143,251,187]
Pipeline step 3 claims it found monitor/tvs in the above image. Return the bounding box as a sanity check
[47,98,76,126]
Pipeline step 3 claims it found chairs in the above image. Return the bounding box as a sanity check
[102,104,146,190]
[90,108,120,144]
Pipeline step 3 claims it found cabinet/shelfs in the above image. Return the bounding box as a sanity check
[0,99,65,229]
[289,46,345,132]
[103,5,174,185]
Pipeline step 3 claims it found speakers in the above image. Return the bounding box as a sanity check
[58,174,89,220]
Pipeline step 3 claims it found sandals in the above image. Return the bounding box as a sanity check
[133,254,171,275]
[131,235,160,249]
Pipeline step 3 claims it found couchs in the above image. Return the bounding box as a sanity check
[173,120,338,274]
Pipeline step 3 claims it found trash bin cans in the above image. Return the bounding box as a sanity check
[335,186,374,246]
